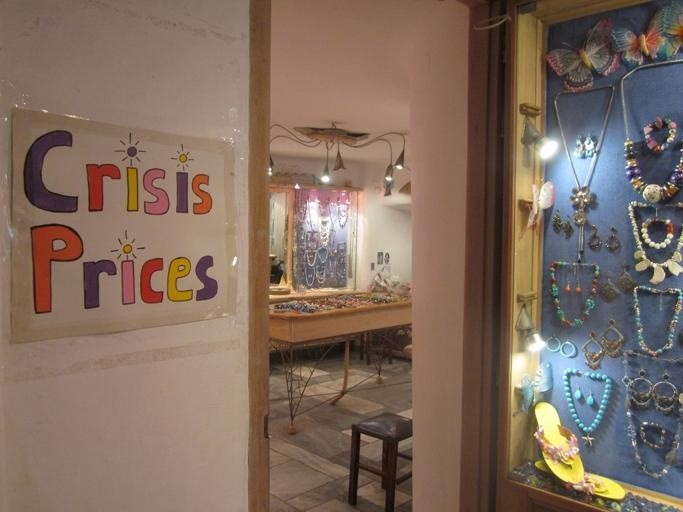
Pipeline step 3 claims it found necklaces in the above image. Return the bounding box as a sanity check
[302,187,351,287]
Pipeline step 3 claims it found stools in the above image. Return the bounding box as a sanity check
[347,411,414,512]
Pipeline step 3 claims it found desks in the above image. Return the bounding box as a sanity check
[269,288,413,436]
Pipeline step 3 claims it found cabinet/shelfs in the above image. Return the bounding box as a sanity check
[269,290,357,354]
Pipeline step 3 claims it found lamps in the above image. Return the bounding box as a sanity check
[522,115,560,165]
[268,120,407,196]
[515,303,547,353]
